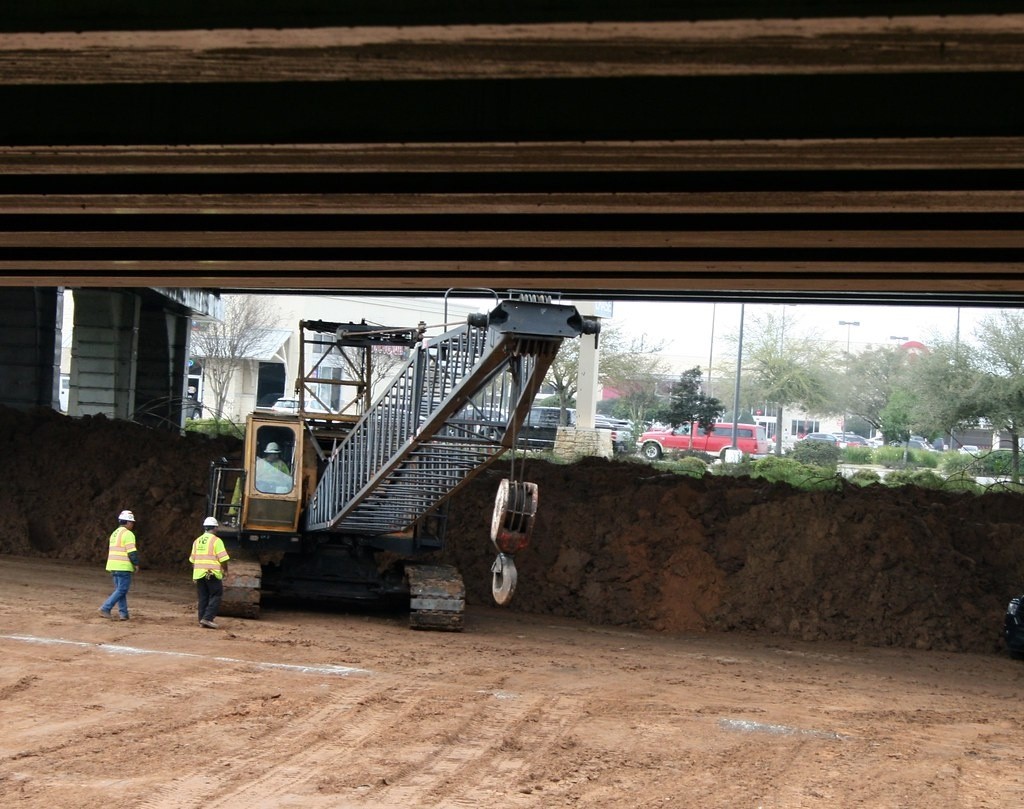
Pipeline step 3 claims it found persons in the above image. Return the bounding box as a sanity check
[96,509,141,620]
[189,516,230,629]
[224,442,289,516]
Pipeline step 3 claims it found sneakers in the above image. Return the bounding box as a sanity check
[97,608,112,618]
[120,615,129,620]
[199,619,218,629]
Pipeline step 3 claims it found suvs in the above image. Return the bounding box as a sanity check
[635,422,768,463]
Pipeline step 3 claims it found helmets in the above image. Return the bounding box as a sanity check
[118,510,135,522]
[203,517,218,526]
[264,442,282,453]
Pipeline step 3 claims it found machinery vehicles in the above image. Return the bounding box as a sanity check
[204,284,603,631]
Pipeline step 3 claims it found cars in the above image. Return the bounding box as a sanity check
[804,429,944,455]
[460,402,634,454]
[271,394,341,424]
[958,445,982,456]
